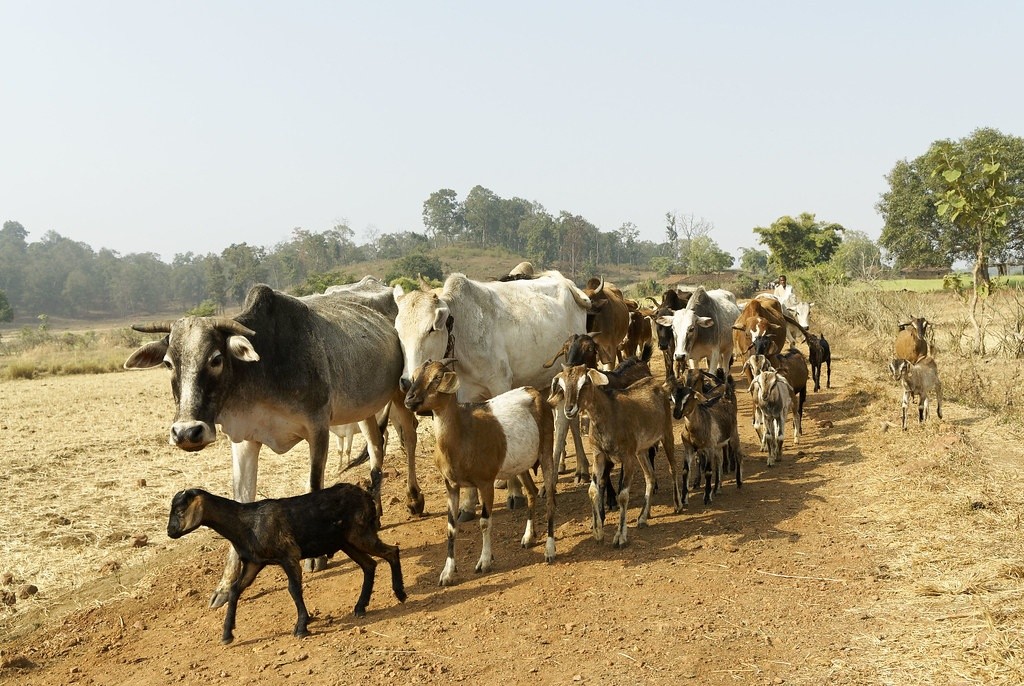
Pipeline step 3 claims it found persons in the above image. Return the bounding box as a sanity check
[774,275,793,298]
[767,282,775,289]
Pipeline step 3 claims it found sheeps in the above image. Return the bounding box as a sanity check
[543,329,686,551]
[398,354,560,589]
[801,331,833,393]
[166,481,410,647]
[736,332,811,470]
[888,314,944,432]
[661,367,746,506]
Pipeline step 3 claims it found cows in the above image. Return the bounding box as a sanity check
[644,279,816,401]
[121,272,432,616]
[390,260,653,524]
